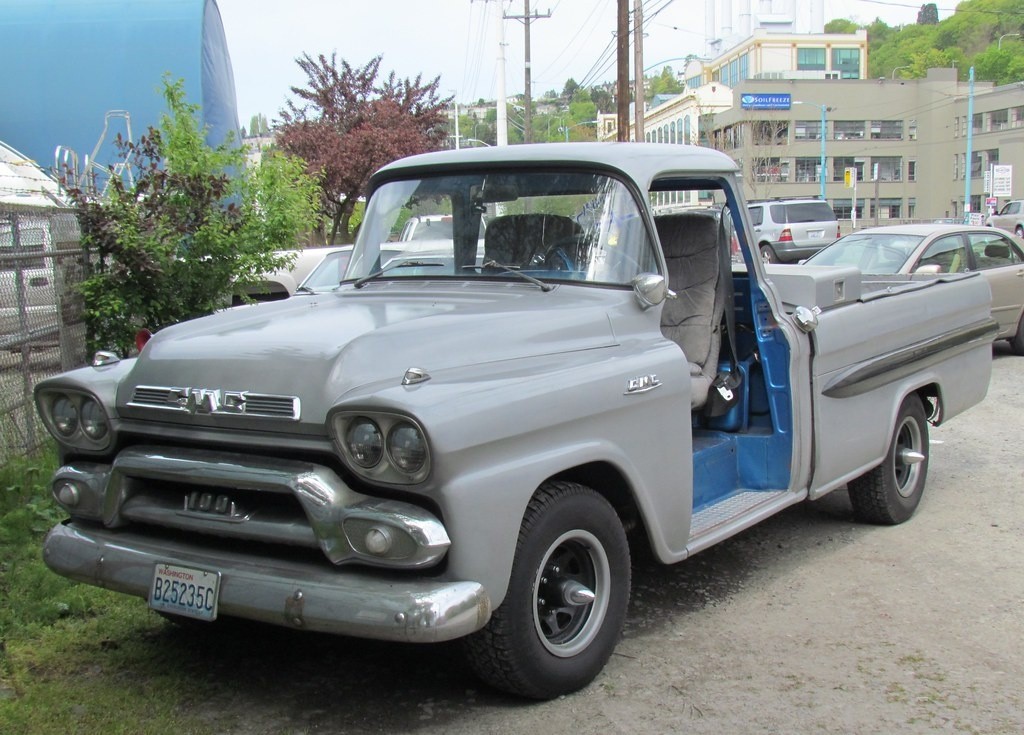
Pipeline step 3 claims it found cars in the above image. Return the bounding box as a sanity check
[985,200,1024,238]
[802,222,1024,356]
[296,211,488,293]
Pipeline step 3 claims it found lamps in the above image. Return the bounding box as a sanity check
[879,77,886,82]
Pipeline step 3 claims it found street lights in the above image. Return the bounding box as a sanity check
[789,100,838,200]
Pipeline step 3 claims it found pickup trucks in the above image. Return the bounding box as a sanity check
[33,140,1001,703]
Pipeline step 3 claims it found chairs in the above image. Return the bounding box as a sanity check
[481,213,587,277]
[976,244,1011,268]
[607,214,731,409]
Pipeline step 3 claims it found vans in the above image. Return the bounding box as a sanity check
[726,198,840,265]
